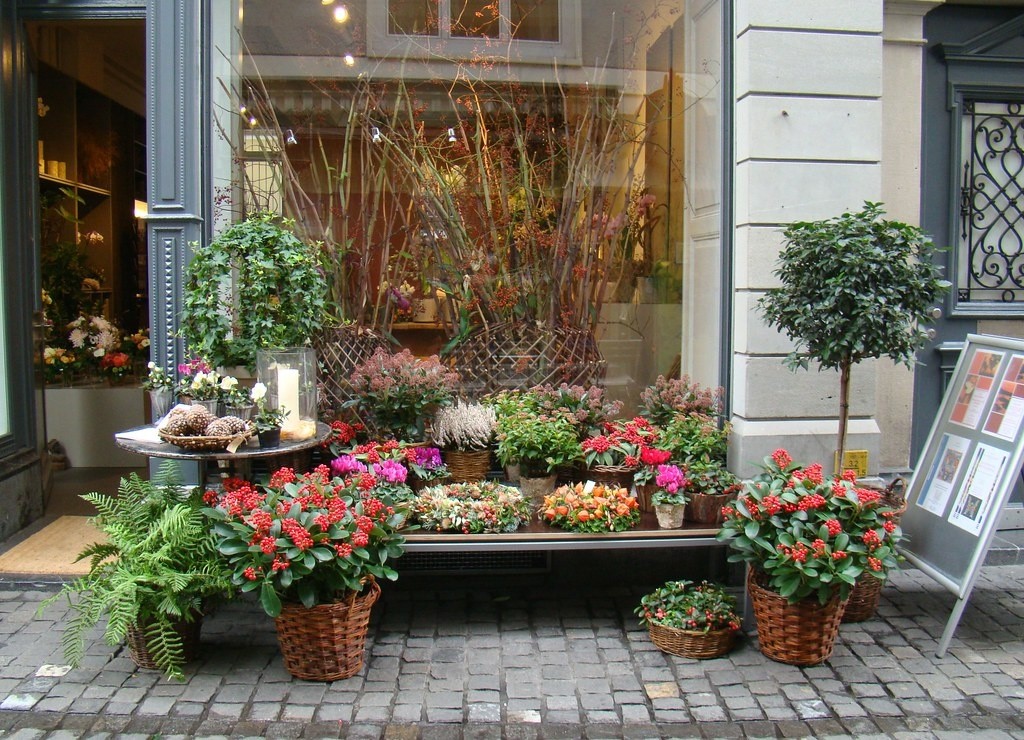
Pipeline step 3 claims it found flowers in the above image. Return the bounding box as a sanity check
[35,231,148,387]
[149,0,911,680]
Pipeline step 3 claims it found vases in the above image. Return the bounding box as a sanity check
[685,488,738,526]
[653,502,686,530]
[224,405,254,421]
[366,463,383,476]
[192,398,218,415]
[412,299,437,322]
[180,394,191,407]
[441,298,462,323]
[502,461,525,483]
[634,276,654,305]
[634,482,660,513]
[590,464,637,495]
[150,389,171,425]
[413,473,444,491]
[517,474,557,504]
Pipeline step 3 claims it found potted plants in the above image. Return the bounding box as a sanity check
[256,408,280,446]
[41,459,232,681]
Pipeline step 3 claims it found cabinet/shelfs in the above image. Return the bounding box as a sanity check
[35,64,112,323]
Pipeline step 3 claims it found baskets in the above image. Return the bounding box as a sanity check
[273,574,381,681]
[158,420,258,452]
[126,597,209,670]
[868,477,907,525]
[646,616,735,658]
[411,473,447,494]
[446,450,490,482]
[381,441,432,468]
[354,500,408,530]
[746,567,849,666]
[589,465,636,496]
[841,564,889,622]
[528,469,576,481]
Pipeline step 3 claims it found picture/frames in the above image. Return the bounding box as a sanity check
[386,0,441,38]
[449,0,501,41]
[509,0,561,44]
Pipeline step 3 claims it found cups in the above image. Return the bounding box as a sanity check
[255,347,317,442]
